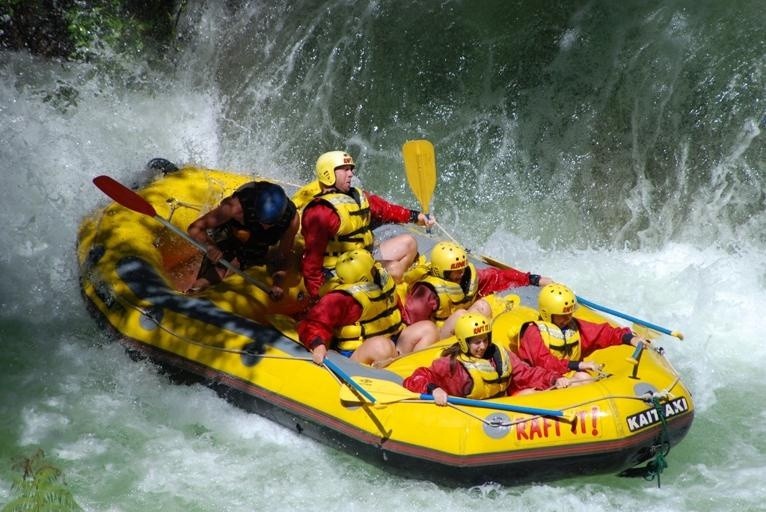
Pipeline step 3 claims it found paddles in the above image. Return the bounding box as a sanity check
[403,139,436,234]
[92,175,272,293]
[340,375,571,418]
[264,312,380,407]
[480,254,683,341]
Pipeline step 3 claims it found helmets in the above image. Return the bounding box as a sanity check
[538,283,578,323]
[454,312,493,354]
[255,183,288,226]
[316,151,356,187]
[431,241,469,279]
[335,248,376,283]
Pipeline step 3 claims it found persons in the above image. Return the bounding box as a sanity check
[293,150,435,296]
[297,248,439,365]
[403,314,571,405]
[509,283,651,397]
[404,241,552,339]
[183,180,300,303]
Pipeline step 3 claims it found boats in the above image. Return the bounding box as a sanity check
[76,166,695,485]
